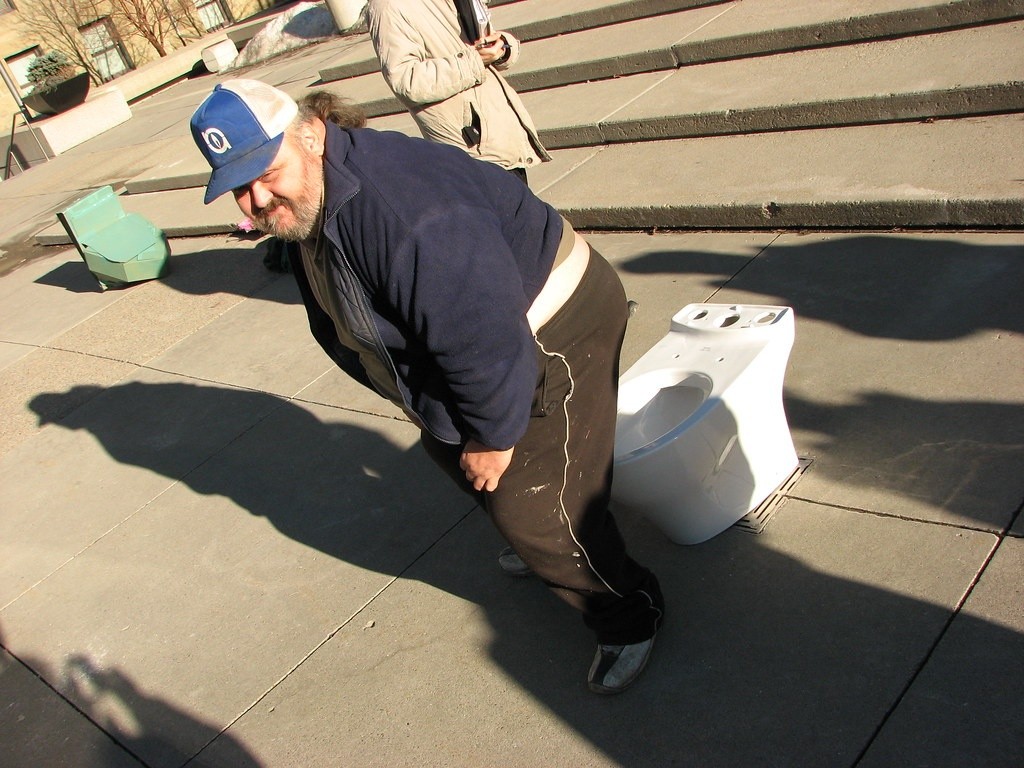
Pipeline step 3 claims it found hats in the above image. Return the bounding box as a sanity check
[189,79,299,204]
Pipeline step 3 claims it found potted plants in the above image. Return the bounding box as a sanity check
[21,50,91,116]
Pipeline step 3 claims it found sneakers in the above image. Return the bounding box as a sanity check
[587,631,656,696]
[498,547,537,577]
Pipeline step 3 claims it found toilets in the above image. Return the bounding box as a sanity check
[611,303,800,548]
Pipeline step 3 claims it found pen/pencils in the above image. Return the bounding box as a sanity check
[476,44,512,49]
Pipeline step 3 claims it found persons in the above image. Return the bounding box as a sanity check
[366,0,554,189]
[189,78,669,695]
